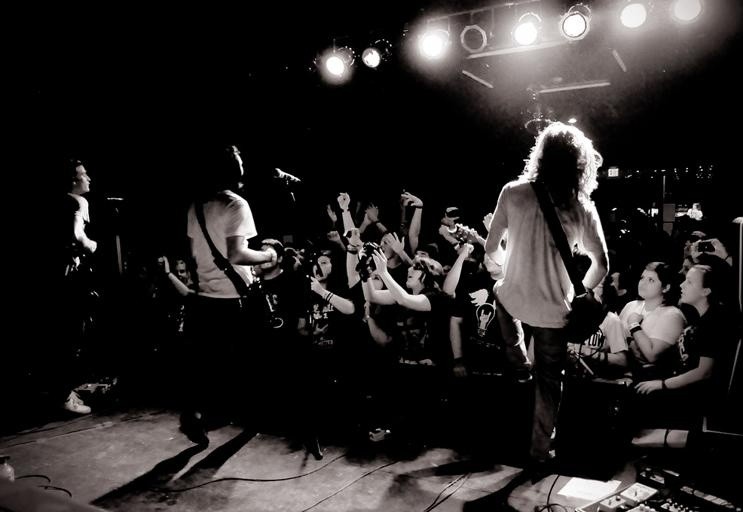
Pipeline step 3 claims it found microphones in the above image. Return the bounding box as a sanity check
[103,194,126,206]
[271,167,302,183]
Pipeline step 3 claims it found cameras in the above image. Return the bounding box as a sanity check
[356,241,384,282]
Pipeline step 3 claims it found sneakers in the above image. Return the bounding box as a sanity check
[179,413,210,447]
[59,392,91,415]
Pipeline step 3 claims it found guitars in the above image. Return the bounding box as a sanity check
[450,223,610,343]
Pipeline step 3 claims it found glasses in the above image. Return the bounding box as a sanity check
[412,262,426,273]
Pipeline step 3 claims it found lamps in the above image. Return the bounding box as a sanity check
[462,55,500,89]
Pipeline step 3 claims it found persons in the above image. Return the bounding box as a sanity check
[567,230,740,468]
[483,121,609,475]
[156,189,497,445]
[176,136,278,448]
[36,154,95,279]
